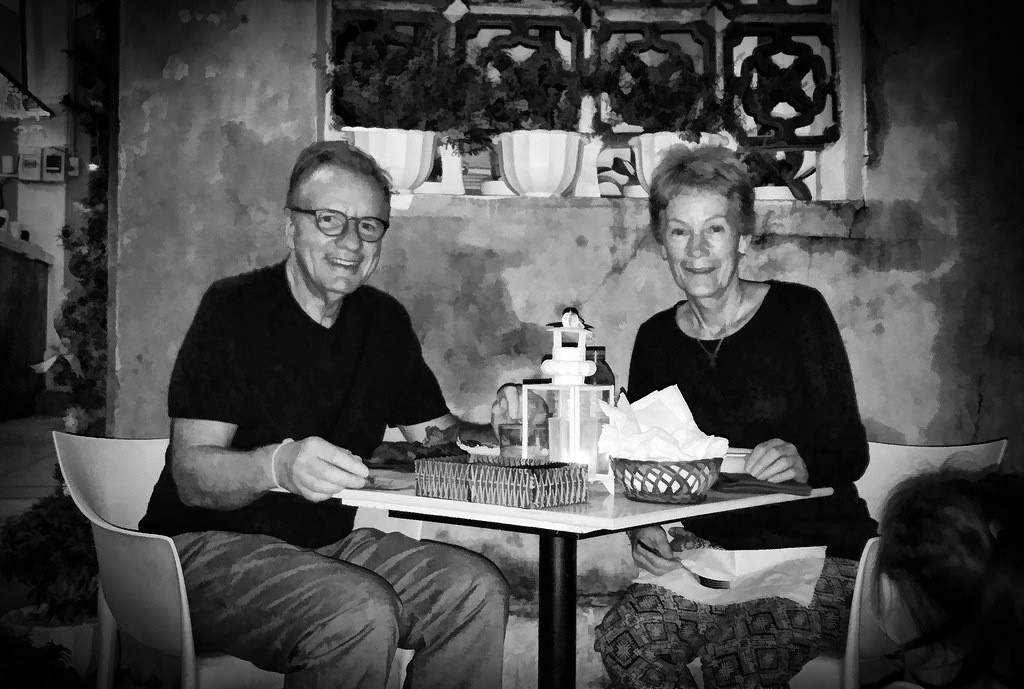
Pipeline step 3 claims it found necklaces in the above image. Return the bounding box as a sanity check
[693,291,742,367]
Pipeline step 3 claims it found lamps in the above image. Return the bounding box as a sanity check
[521,310,615,485]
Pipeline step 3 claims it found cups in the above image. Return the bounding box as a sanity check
[499,424,549,462]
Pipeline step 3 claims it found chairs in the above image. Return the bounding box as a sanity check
[695,438,1009,689]
[53,431,403,689]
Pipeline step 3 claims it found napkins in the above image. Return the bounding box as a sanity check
[632,545,828,608]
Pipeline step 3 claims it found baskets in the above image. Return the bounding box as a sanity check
[608,455,724,505]
[413,451,589,510]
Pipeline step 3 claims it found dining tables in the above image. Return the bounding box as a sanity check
[258,439,835,689]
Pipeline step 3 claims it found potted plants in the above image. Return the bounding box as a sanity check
[310,25,494,198]
[476,35,591,199]
[581,37,729,200]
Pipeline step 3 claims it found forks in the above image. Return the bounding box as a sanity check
[636,540,732,590]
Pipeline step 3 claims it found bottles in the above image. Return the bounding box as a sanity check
[587,346,614,406]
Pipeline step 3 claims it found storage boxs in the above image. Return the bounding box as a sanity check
[413,452,589,509]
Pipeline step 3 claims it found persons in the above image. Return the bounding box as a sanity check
[138,141,547,688]
[869,451,1024,688]
[597,145,879,688]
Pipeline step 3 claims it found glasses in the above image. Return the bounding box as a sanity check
[285,208,389,244]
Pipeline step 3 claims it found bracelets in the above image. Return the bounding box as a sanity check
[270,437,294,493]
